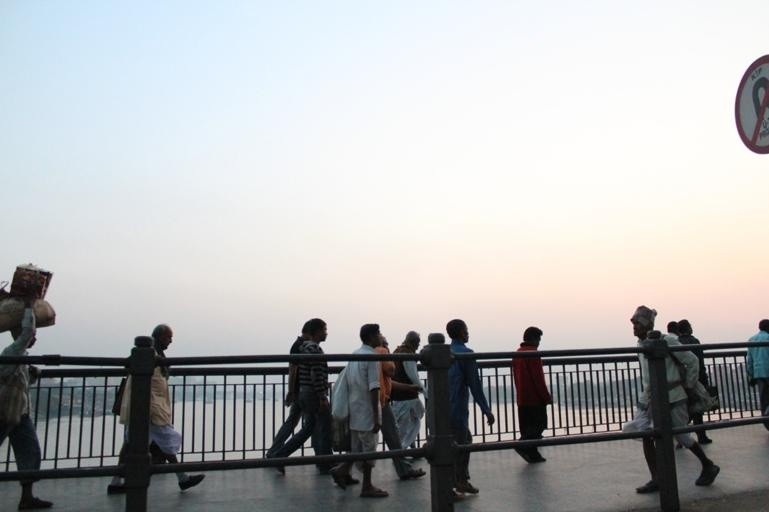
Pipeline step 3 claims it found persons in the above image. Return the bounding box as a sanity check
[512,325,552,463]
[625,304,719,492]
[744,318,769,432]
[0,296,56,511]
[667,318,717,449]
[106,325,205,495]
[263,319,495,501]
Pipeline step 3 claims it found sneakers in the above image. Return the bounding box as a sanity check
[267,451,285,474]
[19,496,52,509]
[400,468,426,480]
[699,437,712,444]
[635,479,658,493]
[108,484,126,495]
[360,487,388,497]
[179,474,204,490]
[515,446,545,463]
[319,464,358,490]
[450,481,480,501]
[695,464,720,486]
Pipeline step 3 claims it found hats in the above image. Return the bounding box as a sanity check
[674,320,692,334]
[630,306,657,330]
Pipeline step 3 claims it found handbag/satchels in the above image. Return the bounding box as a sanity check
[329,422,351,451]
[706,387,719,408]
[112,377,127,414]
[688,385,713,415]
[0,280,55,333]
[331,368,349,421]
